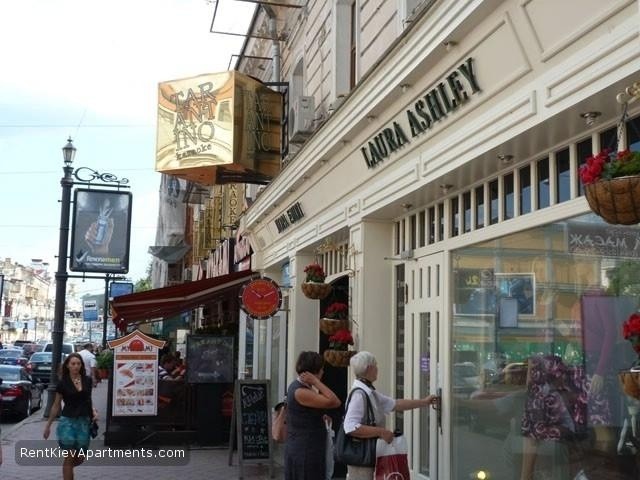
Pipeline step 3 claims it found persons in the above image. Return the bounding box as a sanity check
[520,248,618,480]
[343,351,438,480]
[78,343,98,389]
[158,350,187,382]
[282,351,342,480]
[84,218,115,257]
[43,351,99,480]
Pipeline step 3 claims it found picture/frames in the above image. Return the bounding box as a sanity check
[454,271,536,317]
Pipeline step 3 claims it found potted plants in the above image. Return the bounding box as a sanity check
[96,346,111,379]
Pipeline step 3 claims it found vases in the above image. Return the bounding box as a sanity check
[302,283,332,299]
[320,318,345,335]
[324,349,357,367]
[583,173,640,225]
[617,369,640,399]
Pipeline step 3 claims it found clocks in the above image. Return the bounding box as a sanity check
[237,276,282,320]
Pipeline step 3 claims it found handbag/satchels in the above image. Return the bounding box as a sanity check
[90,420,98,439]
[271,406,288,445]
[334,421,377,468]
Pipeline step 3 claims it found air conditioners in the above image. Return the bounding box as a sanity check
[288,96,316,142]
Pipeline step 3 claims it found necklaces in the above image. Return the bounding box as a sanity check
[69,373,82,385]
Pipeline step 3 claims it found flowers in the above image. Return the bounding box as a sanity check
[577,151,640,184]
[328,329,354,351]
[323,302,348,320]
[622,312,640,339]
[304,264,326,283]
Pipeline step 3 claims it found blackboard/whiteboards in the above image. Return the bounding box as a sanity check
[235,379,273,464]
[186,335,234,384]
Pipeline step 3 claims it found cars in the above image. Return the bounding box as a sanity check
[452,360,528,435]
[0,331,116,420]
